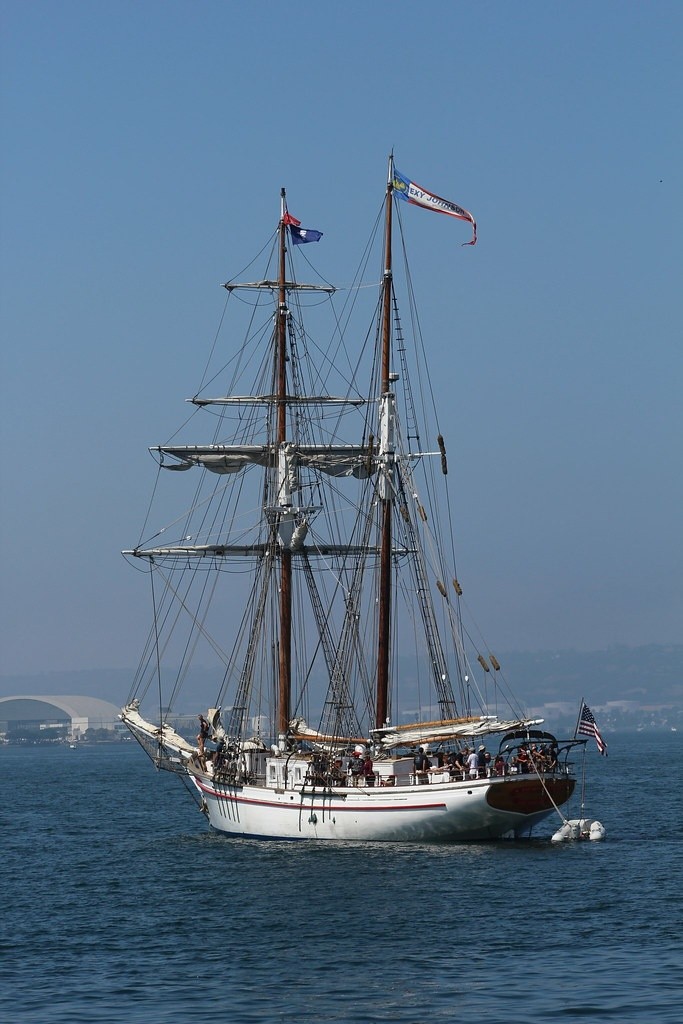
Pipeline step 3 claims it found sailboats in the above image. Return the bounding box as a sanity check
[115,144,590,844]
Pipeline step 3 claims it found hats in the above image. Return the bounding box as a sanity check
[478,745,485,751]
[469,747,476,751]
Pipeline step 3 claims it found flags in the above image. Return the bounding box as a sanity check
[290,223,324,246]
[577,701,609,758]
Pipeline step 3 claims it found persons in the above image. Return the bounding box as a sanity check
[197,713,209,757]
[413,744,505,780]
[511,743,559,774]
[335,752,376,788]
[211,736,224,767]
